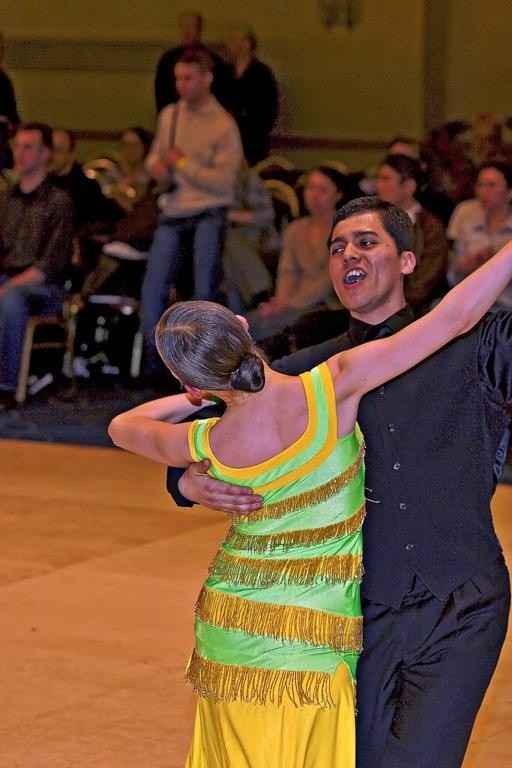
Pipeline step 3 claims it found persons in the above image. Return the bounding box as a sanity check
[2,67,19,170]
[164,194,512,768]
[142,9,282,396]
[2,120,151,410]
[107,232,512,768]
[261,115,512,315]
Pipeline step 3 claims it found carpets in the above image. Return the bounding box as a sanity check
[3,383,511,485]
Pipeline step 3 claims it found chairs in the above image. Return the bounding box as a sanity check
[256,151,349,219]
[14,236,81,402]
